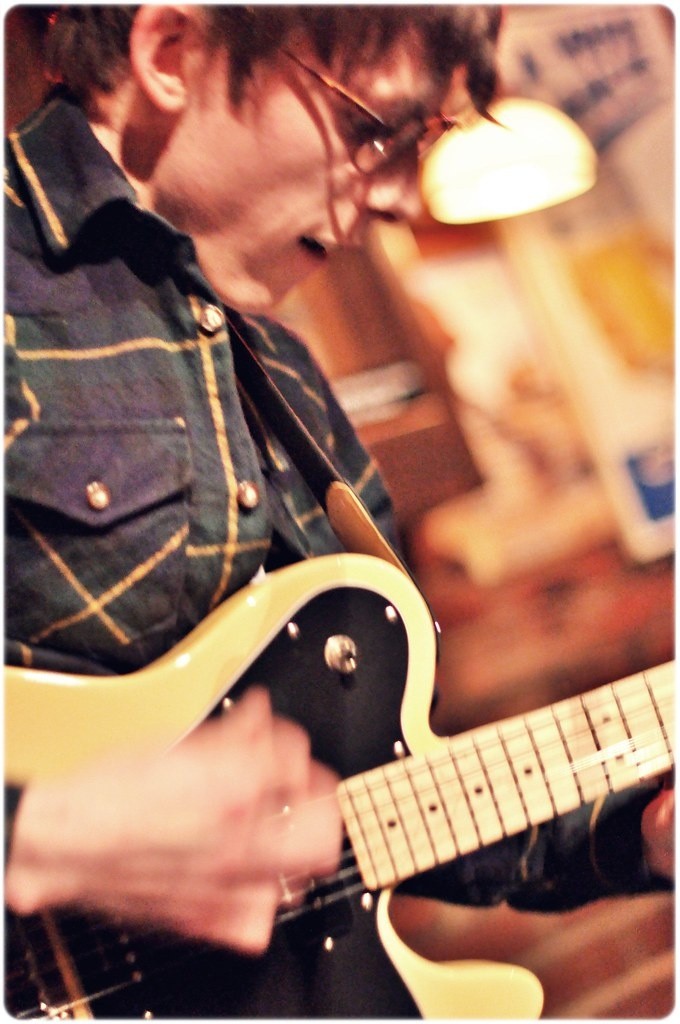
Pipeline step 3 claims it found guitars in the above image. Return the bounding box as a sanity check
[4,549,675,1020]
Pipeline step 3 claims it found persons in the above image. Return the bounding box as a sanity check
[5,6,674,955]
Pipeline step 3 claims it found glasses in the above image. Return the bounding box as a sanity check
[281,45,451,177]
